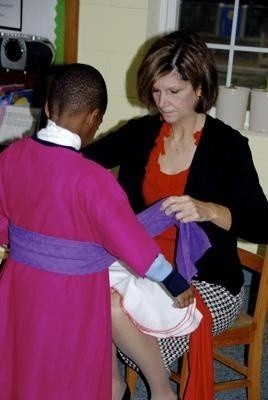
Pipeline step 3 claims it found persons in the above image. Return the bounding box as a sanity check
[0,63,196,399]
[77,30,267,399]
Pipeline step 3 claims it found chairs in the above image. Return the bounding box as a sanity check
[125,243,268,400]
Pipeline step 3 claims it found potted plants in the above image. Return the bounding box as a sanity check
[215,78,251,130]
[251,75,268,133]
